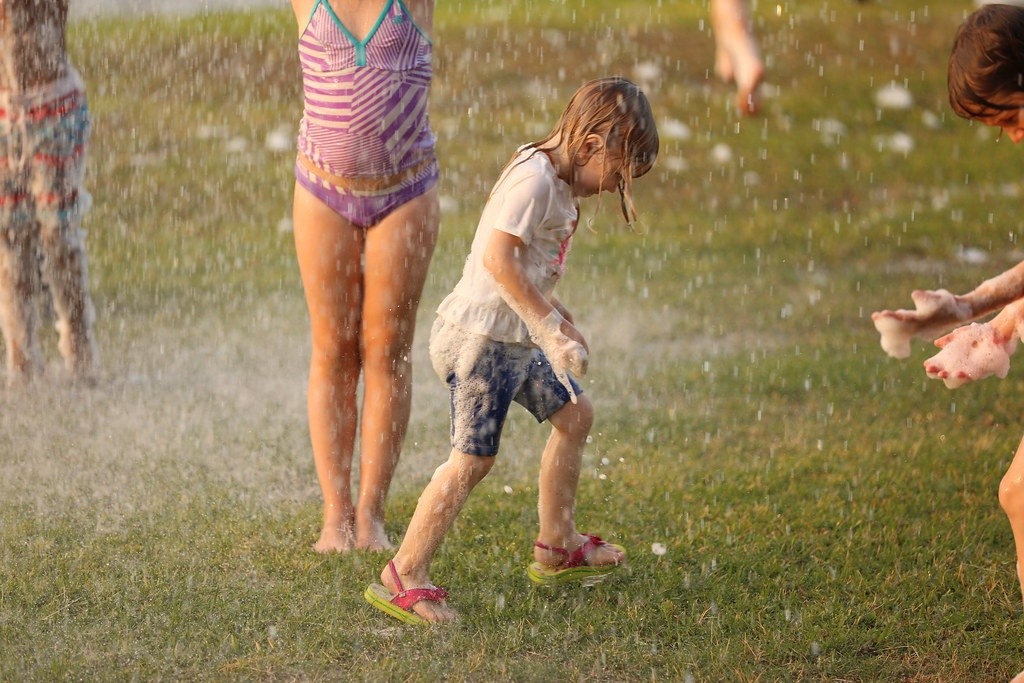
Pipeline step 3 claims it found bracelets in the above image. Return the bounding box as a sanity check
[530,308,565,345]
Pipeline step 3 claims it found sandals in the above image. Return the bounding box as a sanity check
[363,559,461,628]
[527,533,626,585]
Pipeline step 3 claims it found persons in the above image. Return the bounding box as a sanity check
[285,1,442,550]
[866,1,1024,609]
[368,76,659,630]
[701,1,765,116]
[0,0,104,392]
